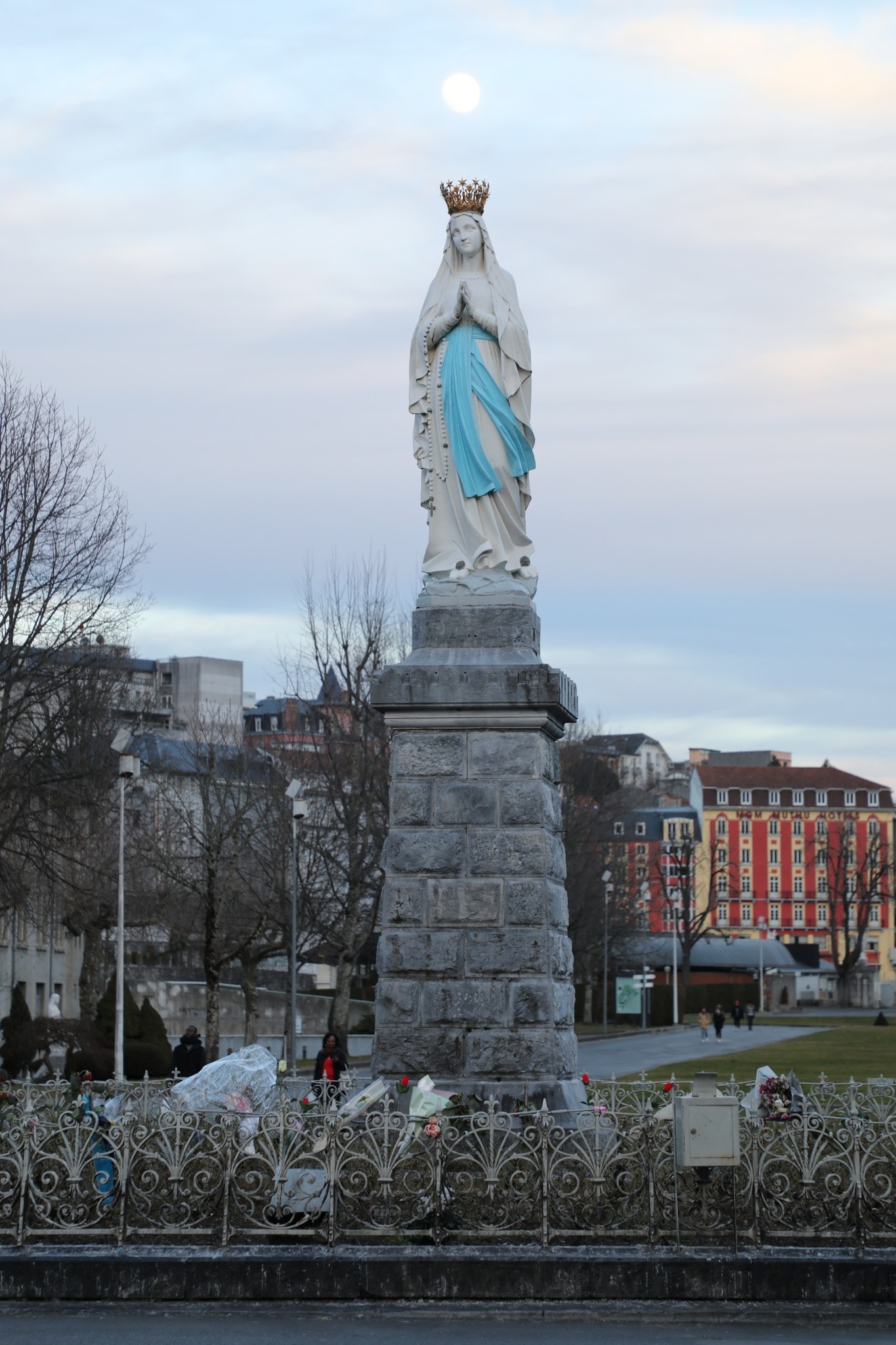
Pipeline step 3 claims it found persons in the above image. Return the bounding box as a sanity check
[310,1031,350,1111]
[172,1025,207,1079]
[745,1003,755,1031]
[698,1007,710,1042]
[410,178,537,581]
[712,1005,725,1042]
[730,1000,744,1030]
[48,993,62,1020]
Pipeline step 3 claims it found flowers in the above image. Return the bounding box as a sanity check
[424,1115,441,1138]
[393,1076,409,1112]
[269,1059,288,1094]
[663,1082,673,1093]
[299,1096,319,1115]
[514,1099,539,1152]
[0,1091,18,1108]
[341,1095,371,1119]
[61,1070,110,1121]
[758,1073,847,1155]
[582,1073,590,1086]
[580,1095,606,1114]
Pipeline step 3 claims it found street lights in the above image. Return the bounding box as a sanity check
[284,779,311,1079]
[107,729,142,1081]
[756,916,767,1014]
[670,890,680,1025]
[602,870,615,1036]
[664,965,671,987]
